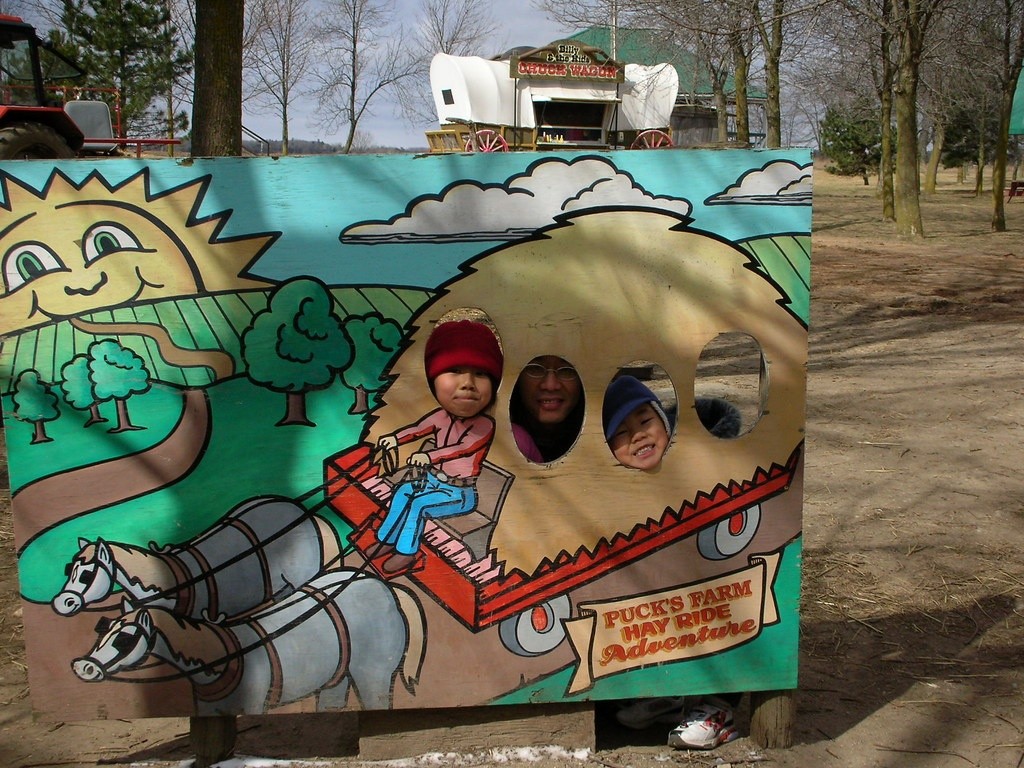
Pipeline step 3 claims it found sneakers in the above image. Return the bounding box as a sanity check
[614,698,682,729]
[668,705,739,750]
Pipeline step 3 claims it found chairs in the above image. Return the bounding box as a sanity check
[63,101,117,153]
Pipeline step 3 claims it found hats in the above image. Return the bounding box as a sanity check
[425,319,503,383]
[603,375,670,442]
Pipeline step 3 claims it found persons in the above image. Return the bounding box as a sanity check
[425,319,503,418]
[510,356,584,464]
[603,375,741,749]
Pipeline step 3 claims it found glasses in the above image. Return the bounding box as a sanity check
[523,364,578,381]
